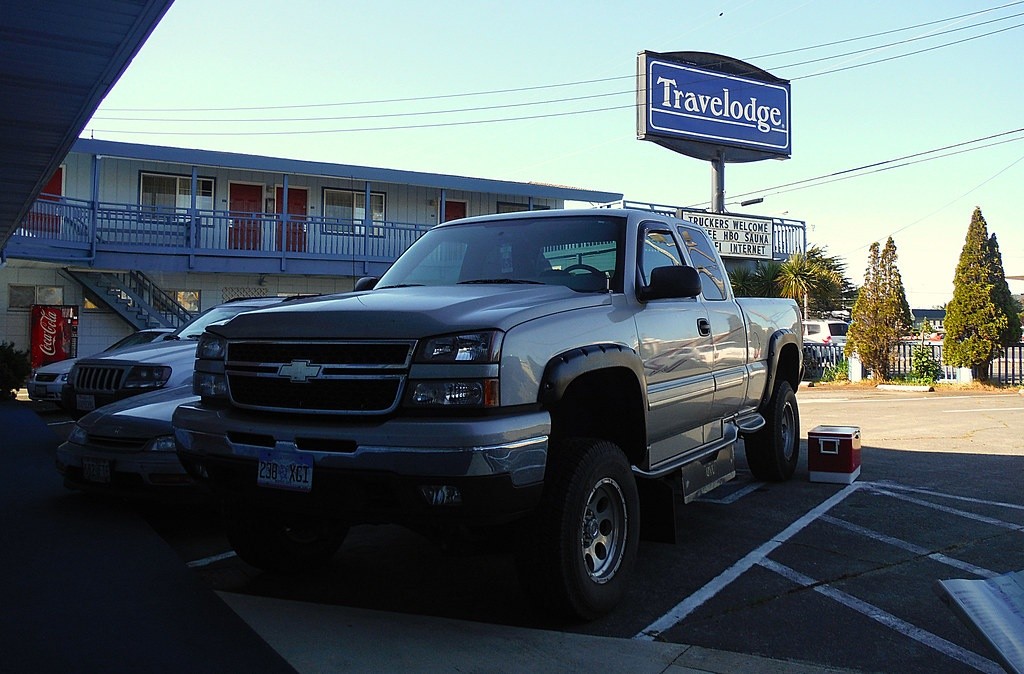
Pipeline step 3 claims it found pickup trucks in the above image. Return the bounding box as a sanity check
[172,207,806,614]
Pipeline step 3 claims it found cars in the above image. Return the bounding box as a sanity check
[795,317,857,381]
[25,327,180,416]
[51,378,223,532]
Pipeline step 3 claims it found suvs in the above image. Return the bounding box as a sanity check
[66,291,327,427]
